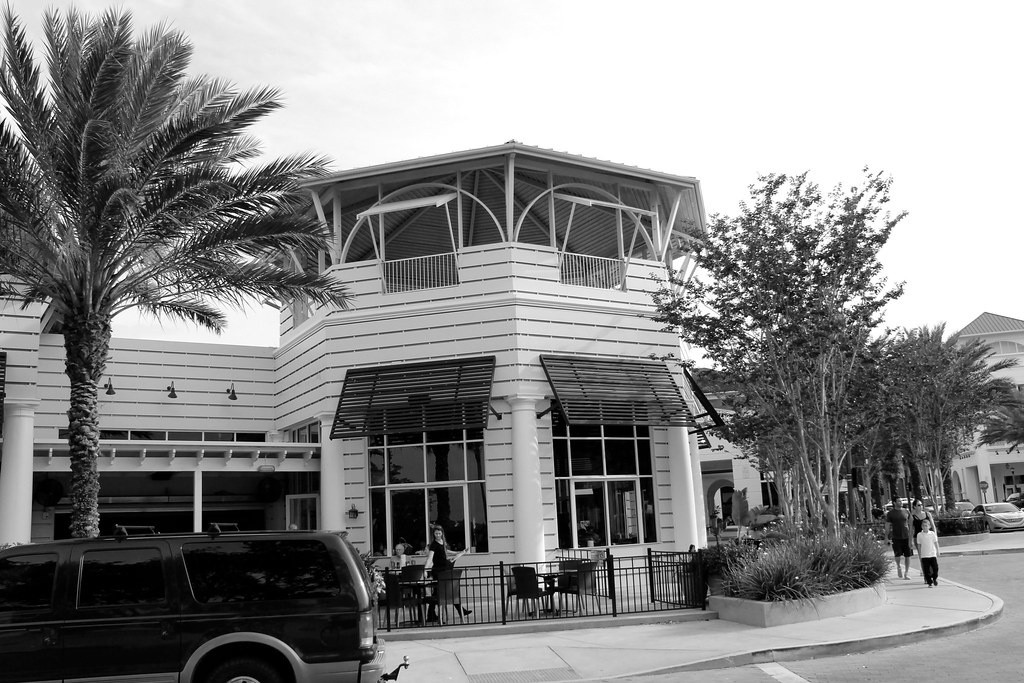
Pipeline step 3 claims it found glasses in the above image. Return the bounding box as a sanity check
[918,504,923,506]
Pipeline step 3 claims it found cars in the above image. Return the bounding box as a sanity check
[969,501,1024,533]
[873,491,1024,524]
[938,501,976,534]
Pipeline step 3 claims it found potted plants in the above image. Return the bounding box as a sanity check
[710,506,722,529]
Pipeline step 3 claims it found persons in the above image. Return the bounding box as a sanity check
[872,504,885,520]
[424,525,472,622]
[917,520,940,587]
[884,496,913,579]
[389,544,411,568]
[907,498,936,575]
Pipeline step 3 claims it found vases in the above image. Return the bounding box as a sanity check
[587,539,595,547]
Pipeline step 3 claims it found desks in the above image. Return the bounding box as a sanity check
[397,579,439,627]
[535,569,578,616]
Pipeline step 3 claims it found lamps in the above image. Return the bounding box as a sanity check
[348,504,359,519]
[258,465,275,472]
[226,383,237,400]
[166,381,177,399]
[104,377,116,395]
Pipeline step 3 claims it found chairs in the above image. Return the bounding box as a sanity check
[502,560,602,621]
[368,564,467,629]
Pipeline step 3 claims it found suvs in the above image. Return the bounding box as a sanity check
[0,521,410,683]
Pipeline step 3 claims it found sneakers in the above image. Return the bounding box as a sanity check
[897,566,903,578]
[903,573,911,580]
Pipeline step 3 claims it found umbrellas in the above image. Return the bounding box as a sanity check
[820,478,871,496]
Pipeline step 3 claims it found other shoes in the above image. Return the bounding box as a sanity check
[458,607,472,618]
[928,583,933,587]
[933,578,938,586]
[427,615,439,622]
